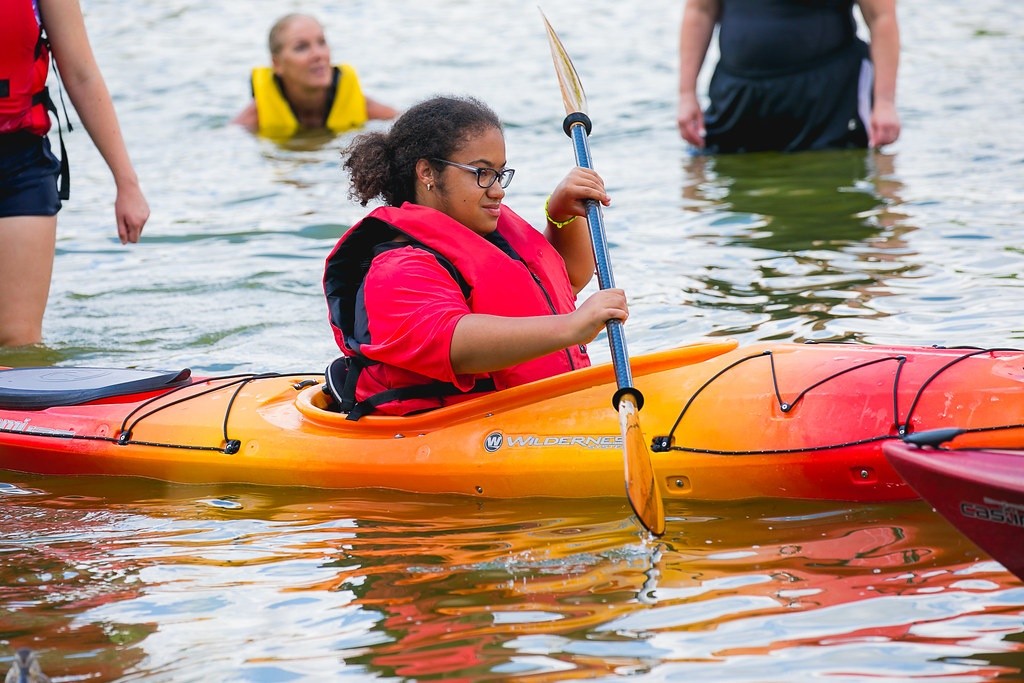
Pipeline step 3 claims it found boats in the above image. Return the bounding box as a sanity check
[0,338,1018,504]
[876,420,1024,581]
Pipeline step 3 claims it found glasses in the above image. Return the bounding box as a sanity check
[430,156,516,189]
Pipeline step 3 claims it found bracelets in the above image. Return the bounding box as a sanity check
[545,194,577,229]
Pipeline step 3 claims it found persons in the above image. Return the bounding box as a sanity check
[233,12,395,138]
[678,0,899,153]
[324,96,628,415]
[0,0,151,347]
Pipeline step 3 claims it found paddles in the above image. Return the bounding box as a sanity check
[537,8,668,540]
